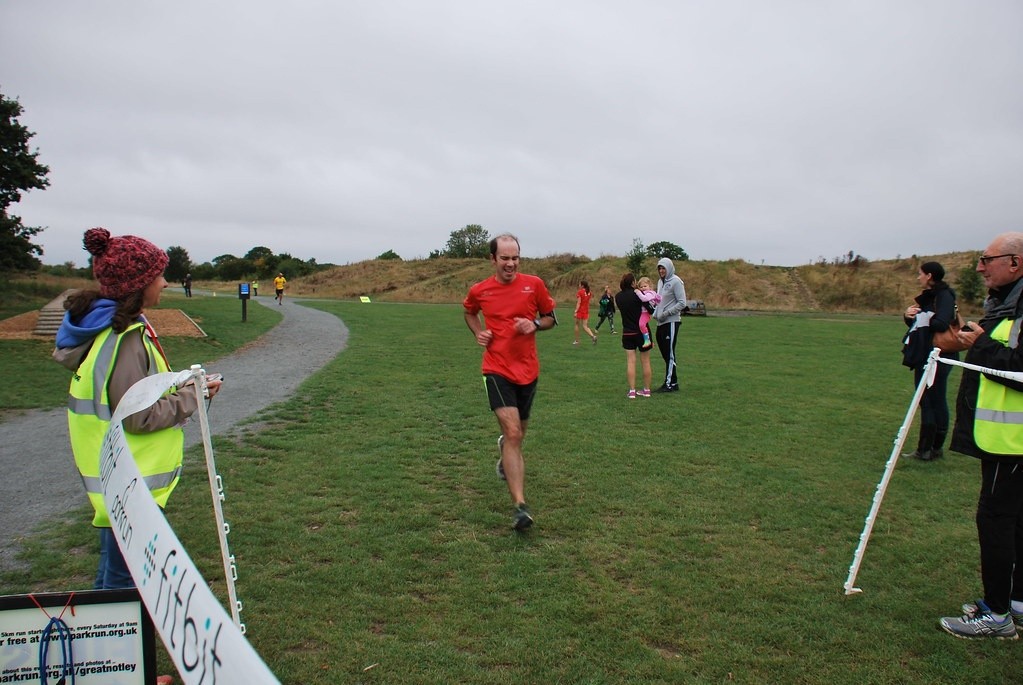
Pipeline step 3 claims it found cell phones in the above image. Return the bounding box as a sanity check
[961,325,973,332]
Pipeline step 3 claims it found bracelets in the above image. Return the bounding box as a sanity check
[534,319,540,331]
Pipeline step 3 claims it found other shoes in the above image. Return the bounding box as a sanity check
[279,303,281,305]
[594,328,598,334]
[275,297,278,300]
[654,384,679,393]
[593,336,597,345]
[901,449,921,460]
[573,340,581,345]
[157,674,172,685]
[610,331,618,334]
[921,447,945,461]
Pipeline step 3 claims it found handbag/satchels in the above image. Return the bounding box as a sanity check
[932,290,969,353]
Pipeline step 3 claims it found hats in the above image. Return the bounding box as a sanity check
[82,228,169,301]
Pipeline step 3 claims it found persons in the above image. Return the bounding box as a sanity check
[182,274,192,297]
[252,277,259,296]
[938,231,1023,641]
[52,225,223,685]
[652,257,686,393]
[573,280,597,345]
[634,277,662,348]
[614,273,655,398]
[901,262,960,460]
[594,288,618,335]
[273,273,288,305]
[463,231,555,529]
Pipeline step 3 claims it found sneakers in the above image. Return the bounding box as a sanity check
[509,502,535,530]
[940,610,1019,640]
[961,594,1023,631]
[495,434,506,480]
[636,388,651,397]
[626,391,638,398]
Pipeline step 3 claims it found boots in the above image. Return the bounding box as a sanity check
[643,332,653,349]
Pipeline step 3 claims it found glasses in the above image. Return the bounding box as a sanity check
[979,253,1017,266]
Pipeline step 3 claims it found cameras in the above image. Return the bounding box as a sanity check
[208,376,224,383]
